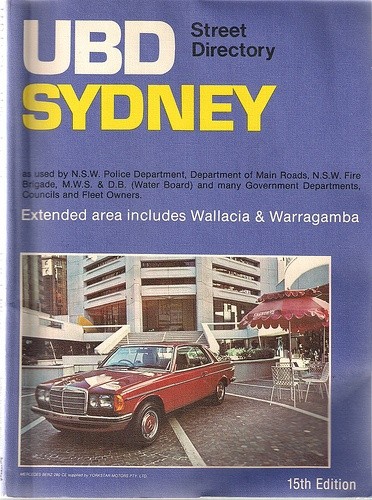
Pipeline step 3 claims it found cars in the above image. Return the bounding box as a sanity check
[31,340,237,447]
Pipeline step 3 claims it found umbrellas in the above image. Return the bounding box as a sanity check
[237,286,331,400]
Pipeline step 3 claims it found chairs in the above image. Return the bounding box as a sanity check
[143,353,160,369]
[176,354,191,370]
[269,361,330,407]
